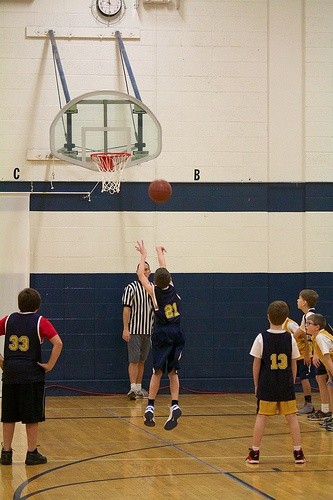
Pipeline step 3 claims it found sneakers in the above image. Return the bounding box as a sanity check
[306,409,332,420]
[128,388,138,401]
[318,415,332,425]
[25,447,48,465]
[143,406,156,427]
[292,447,305,464]
[1,447,16,465]
[163,405,182,430]
[296,402,315,414]
[245,447,260,464]
[137,389,145,396]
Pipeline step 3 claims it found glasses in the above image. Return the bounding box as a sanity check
[305,322,317,326]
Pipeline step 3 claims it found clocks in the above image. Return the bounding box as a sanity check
[97,0,122,17]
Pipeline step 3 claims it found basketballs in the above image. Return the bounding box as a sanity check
[149,180,172,204]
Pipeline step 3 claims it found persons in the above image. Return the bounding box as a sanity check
[281,317,315,414]
[304,313,333,431]
[135,239,185,431]
[297,289,332,421]
[0,288,63,465]
[123,261,155,400]
[246,301,307,463]
